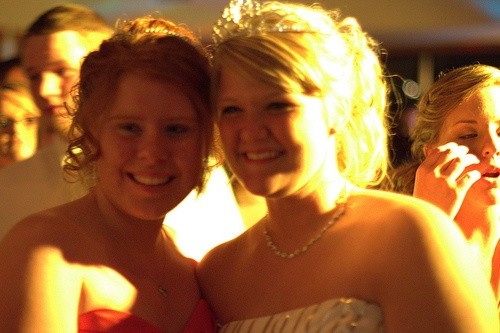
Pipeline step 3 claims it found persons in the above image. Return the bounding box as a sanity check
[0,7,116,242]
[382,63,500,309]
[0,15,215,333]
[195,0,500,333]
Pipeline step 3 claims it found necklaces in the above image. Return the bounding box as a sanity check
[264,197,346,259]
